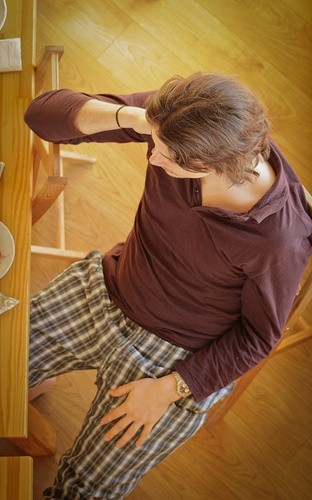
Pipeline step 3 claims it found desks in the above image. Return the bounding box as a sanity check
[0,456,43,500]
[1,0,58,456]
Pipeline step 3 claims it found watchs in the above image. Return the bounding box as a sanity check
[172,371,191,398]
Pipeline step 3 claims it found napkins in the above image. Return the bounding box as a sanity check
[0,37,22,72]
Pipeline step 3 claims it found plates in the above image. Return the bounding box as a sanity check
[0,221,15,280]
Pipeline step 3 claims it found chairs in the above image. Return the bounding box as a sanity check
[32,46,98,259]
[204,184,312,428]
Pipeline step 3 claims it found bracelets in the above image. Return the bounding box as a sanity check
[116,105,126,128]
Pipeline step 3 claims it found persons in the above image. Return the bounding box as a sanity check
[24,70,312,500]
